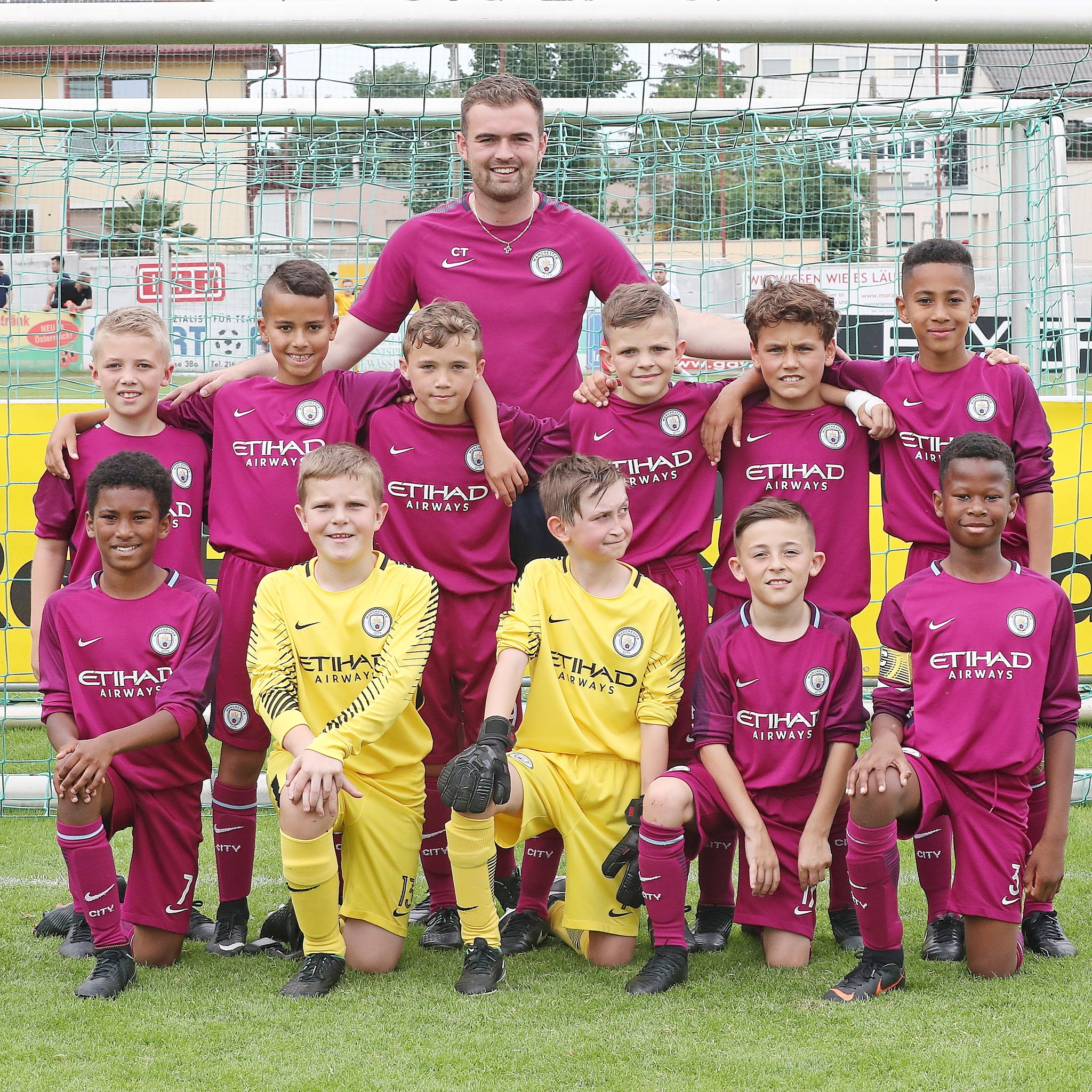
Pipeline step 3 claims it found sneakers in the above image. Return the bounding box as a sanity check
[73,944,137,1001]
[260,897,304,954]
[453,938,507,996]
[647,903,733,951]
[408,865,567,911]
[32,874,217,958]
[822,955,906,1003]
[280,953,347,997]
[626,946,690,995]
[419,906,463,951]
[922,912,967,963]
[829,910,865,957]
[500,912,554,955]
[1022,910,1079,959]
[208,905,249,957]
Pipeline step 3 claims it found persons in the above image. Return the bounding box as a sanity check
[151,73,853,581]
[0,257,1091,1003]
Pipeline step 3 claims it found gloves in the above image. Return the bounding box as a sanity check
[602,796,646,908]
[437,715,518,813]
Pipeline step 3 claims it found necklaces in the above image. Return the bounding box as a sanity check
[471,191,535,254]
